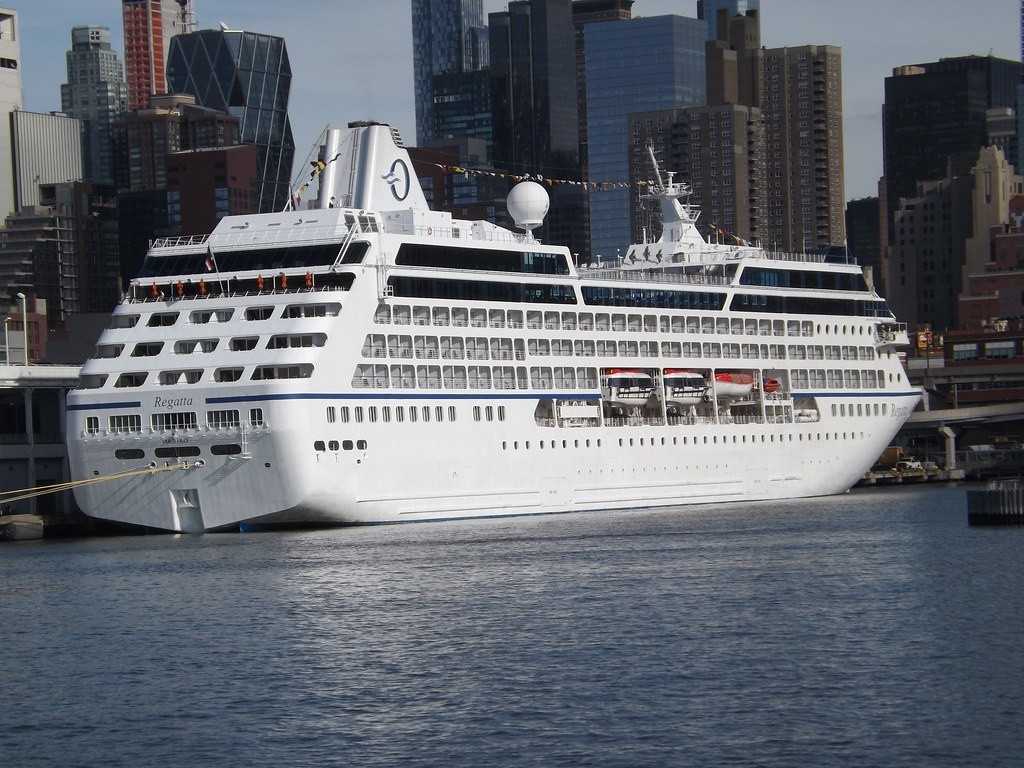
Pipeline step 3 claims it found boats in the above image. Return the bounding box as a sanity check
[706,375,755,396]
[757,379,779,390]
[654,373,710,406]
[602,370,655,404]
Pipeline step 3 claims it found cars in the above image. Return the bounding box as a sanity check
[896,456,921,470]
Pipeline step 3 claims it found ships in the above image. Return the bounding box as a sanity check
[61,121,928,536]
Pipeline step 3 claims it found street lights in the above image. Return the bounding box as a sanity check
[17,294,28,366]
[5,317,13,364]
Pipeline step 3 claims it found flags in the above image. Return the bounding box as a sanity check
[204,247,214,270]
[437,164,656,193]
[708,223,754,248]
[293,153,341,206]
